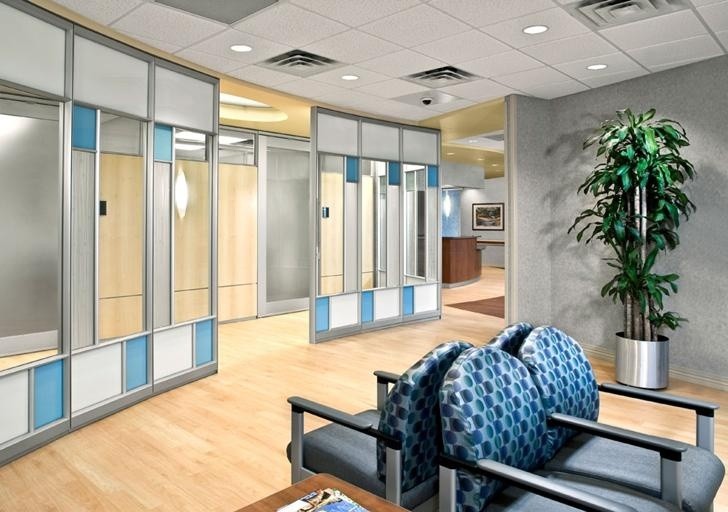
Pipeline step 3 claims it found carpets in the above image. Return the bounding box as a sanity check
[442,295,503,319]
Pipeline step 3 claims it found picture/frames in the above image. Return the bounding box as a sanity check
[471,203,504,231]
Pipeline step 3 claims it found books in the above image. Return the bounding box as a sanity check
[275,486,371,511]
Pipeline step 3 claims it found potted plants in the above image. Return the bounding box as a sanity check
[563,106,696,392]
